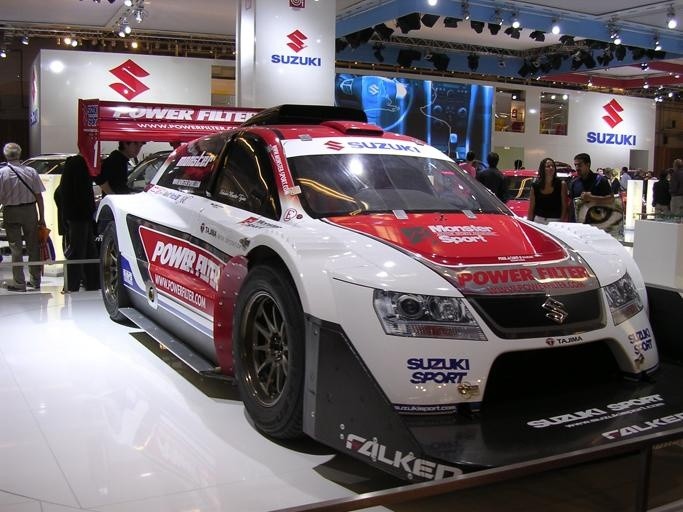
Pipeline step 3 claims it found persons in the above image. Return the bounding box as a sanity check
[597,159,683,218]
[452,151,476,199]
[0,142,46,292]
[475,152,505,202]
[568,153,615,223]
[527,157,568,222]
[53,141,102,294]
[93,141,146,198]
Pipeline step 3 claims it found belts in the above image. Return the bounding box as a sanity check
[6,202,32,207]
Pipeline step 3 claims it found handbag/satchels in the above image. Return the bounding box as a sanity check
[37,225,55,265]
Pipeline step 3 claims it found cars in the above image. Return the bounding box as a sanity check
[431,159,490,193]
[495,169,576,218]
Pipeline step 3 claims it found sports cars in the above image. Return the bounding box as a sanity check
[76,99,681,485]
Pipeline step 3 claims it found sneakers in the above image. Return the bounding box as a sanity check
[6,285,25,292]
[26,282,40,290]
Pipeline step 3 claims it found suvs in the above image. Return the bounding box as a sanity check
[0,154,137,258]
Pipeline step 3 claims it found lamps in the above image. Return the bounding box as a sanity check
[643,80,673,103]
[0,0,151,59]
